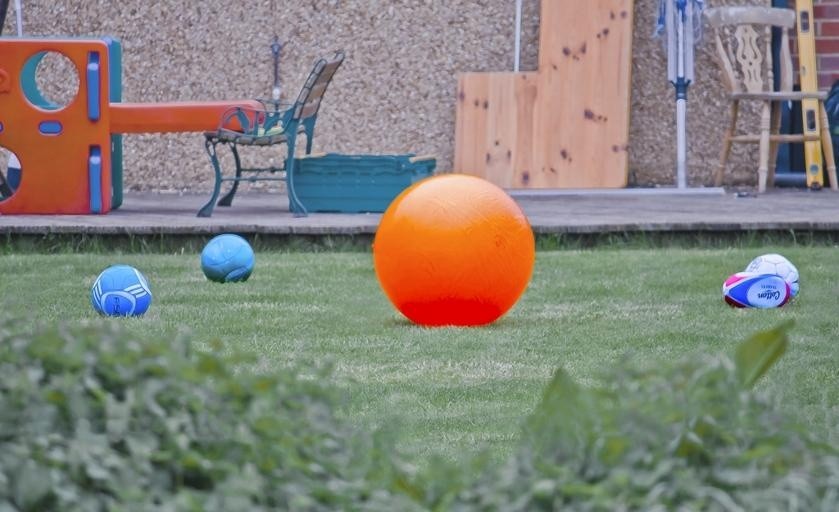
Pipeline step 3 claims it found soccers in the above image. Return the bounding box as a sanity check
[746,253,799,299]
[201,232,255,283]
[94,267,151,319]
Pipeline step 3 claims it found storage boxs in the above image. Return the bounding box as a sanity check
[283,151,436,215]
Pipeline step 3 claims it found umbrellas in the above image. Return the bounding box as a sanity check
[651,1,723,189]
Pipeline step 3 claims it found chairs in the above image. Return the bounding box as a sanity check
[704,7,839,194]
[196,51,346,217]
